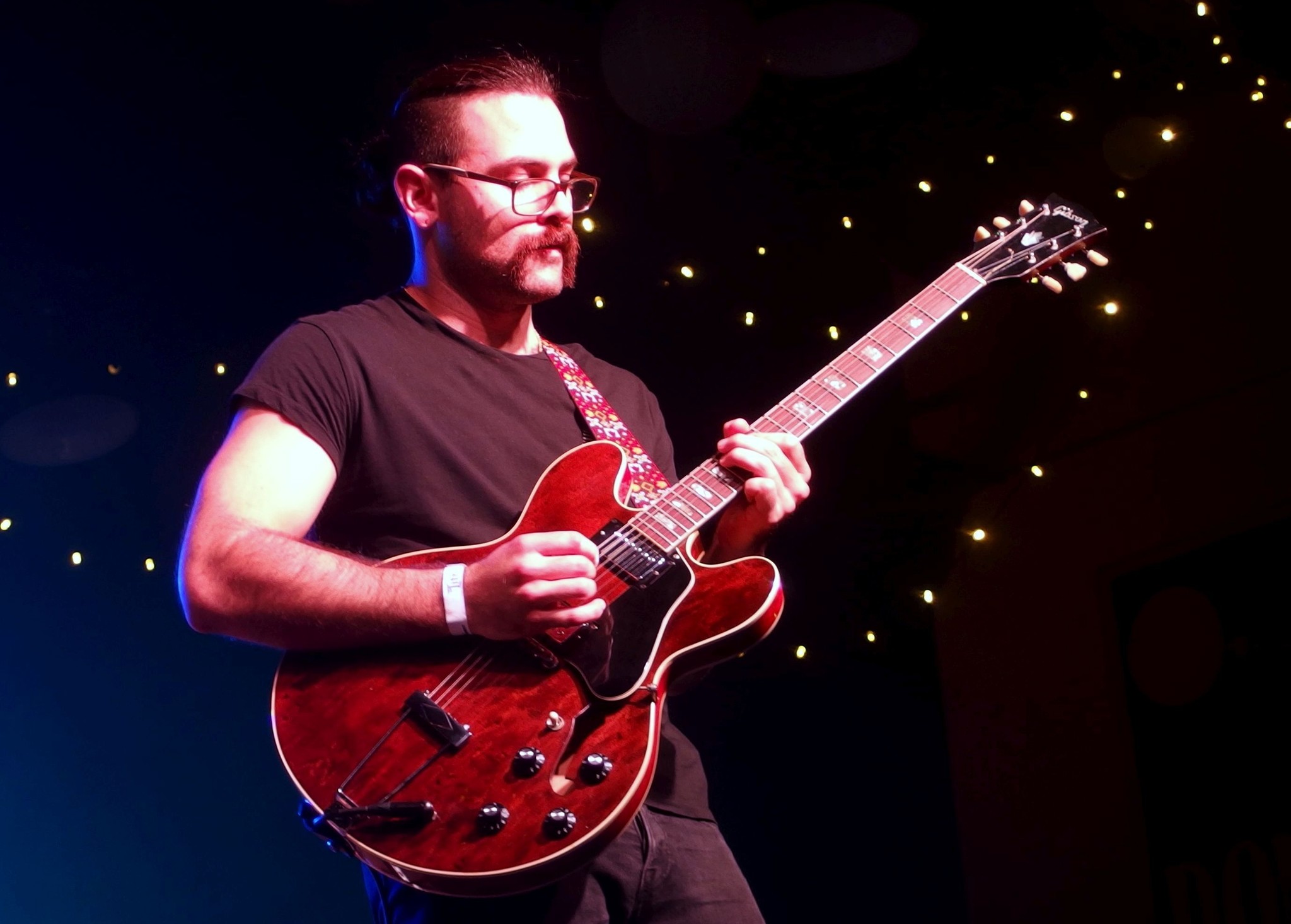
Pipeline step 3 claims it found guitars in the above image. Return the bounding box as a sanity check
[270,200,1112,897]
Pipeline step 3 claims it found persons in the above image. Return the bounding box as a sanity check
[176,60,815,924]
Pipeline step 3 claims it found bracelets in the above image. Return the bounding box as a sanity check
[441,563,470,639]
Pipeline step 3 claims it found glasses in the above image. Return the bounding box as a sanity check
[423,163,601,216]
